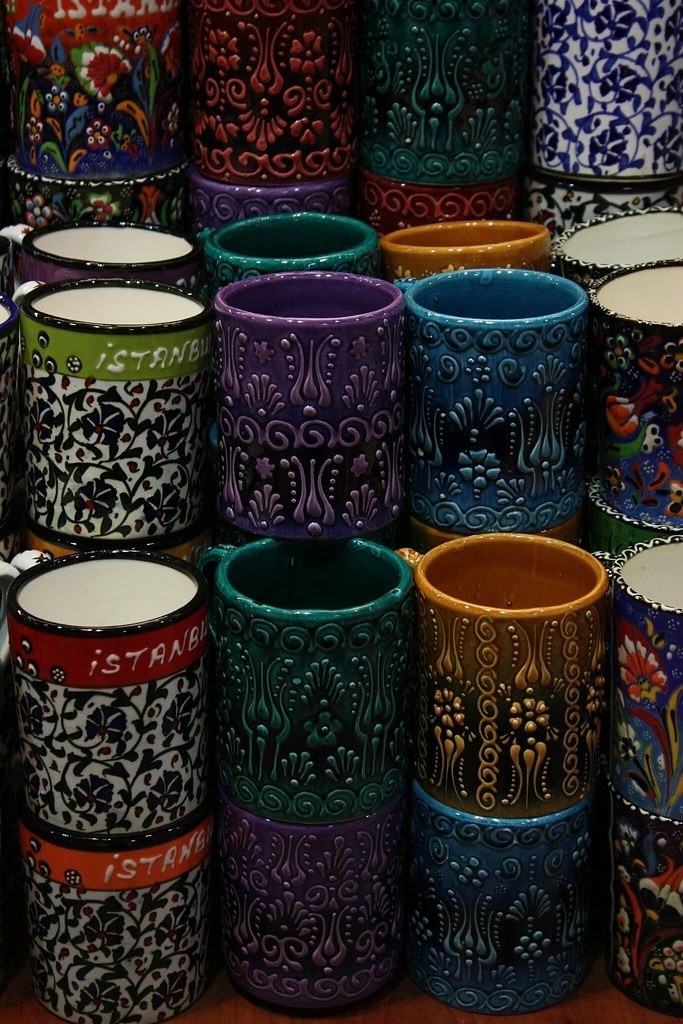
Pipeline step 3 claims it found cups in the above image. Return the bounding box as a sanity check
[1,1,683,1024]
